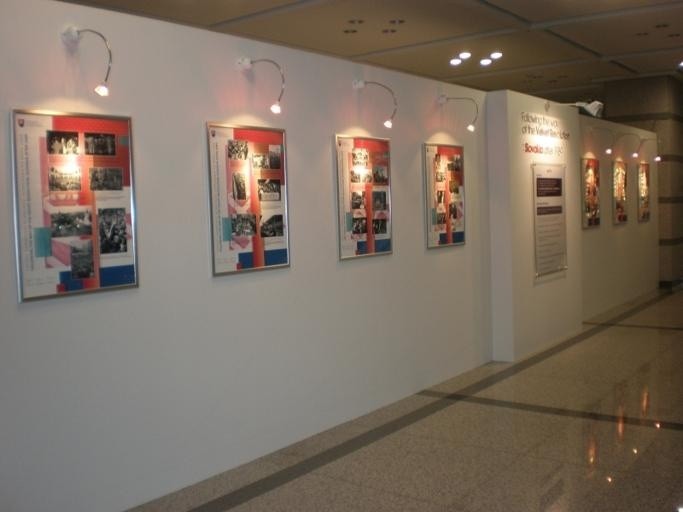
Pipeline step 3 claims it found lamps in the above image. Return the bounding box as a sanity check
[544,100,604,118]
[65,26,113,96]
[438,95,480,131]
[352,80,398,129]
[590,127,664,163]
[239,56,286,116]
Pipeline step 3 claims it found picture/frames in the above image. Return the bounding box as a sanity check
[333,133,396,260]
[8,110,140,302]
[580,158,650,228]
[206,121,292,276]
[422,142,468,250]
